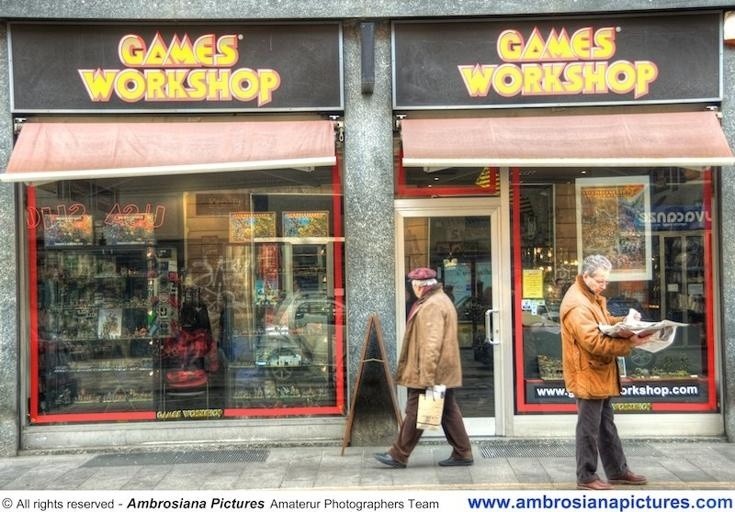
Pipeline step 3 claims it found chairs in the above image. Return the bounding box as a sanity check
[160,325,218,414]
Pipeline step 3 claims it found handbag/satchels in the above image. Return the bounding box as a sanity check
[416,386,444,432]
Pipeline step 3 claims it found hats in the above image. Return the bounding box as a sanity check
[407,268,436,282]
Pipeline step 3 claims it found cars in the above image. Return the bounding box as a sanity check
[256,292,347,384]
[34,299,153,405]
[470,294,660,373]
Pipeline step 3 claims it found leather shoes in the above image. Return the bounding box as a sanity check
[608,470,647,485]
[577,479,613,490]
[439,457,474,466]
[374,452,406,468]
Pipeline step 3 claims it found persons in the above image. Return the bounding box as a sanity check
[370,267,475,467]
[562,254,647,489]
